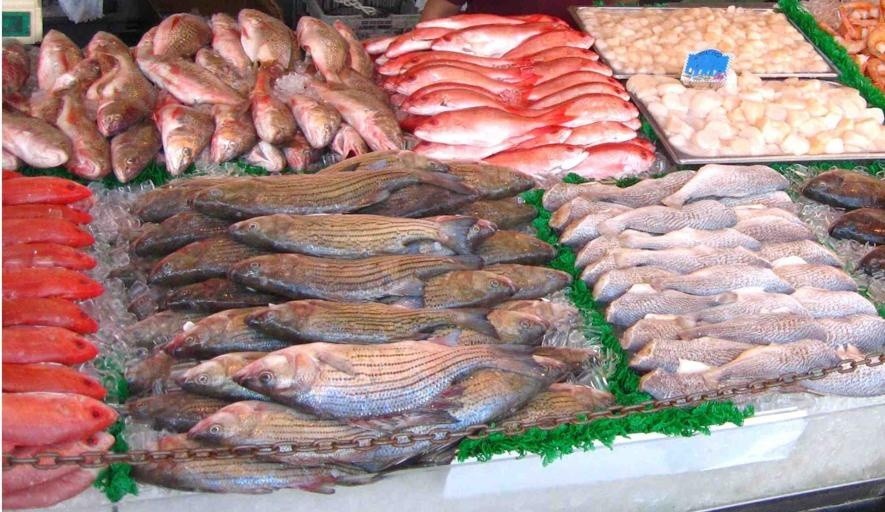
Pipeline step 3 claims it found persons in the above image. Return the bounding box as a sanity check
[417,0,588,33]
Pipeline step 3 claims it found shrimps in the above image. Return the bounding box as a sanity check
[820,1,884,91]
